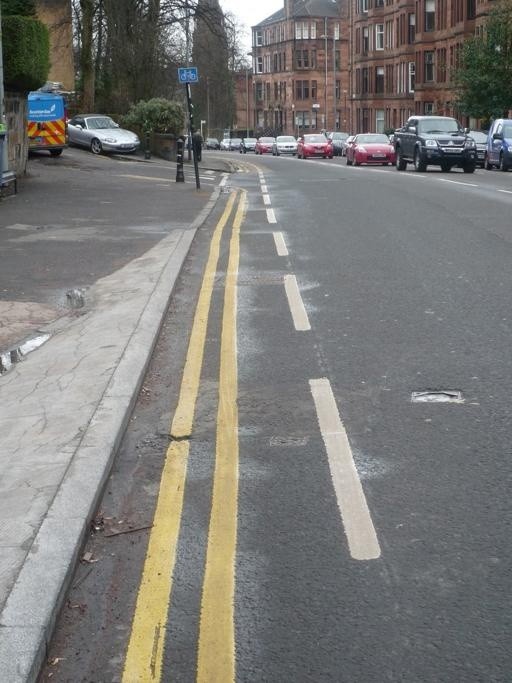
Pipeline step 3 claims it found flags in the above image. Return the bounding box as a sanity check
[201,119,206,123]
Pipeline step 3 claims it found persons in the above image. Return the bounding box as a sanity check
[193,130,203,161]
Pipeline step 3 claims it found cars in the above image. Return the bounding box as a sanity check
[65,108,142,158]
[344,130,398,167]
[184,128,397,158]
[483,115,511,173]
[466,130,489,167]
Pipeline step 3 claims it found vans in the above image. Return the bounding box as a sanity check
[1,77,75,158]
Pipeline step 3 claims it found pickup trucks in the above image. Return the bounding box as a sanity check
[390,113,478,175]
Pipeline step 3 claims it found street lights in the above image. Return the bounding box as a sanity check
[319,31,330,128]
